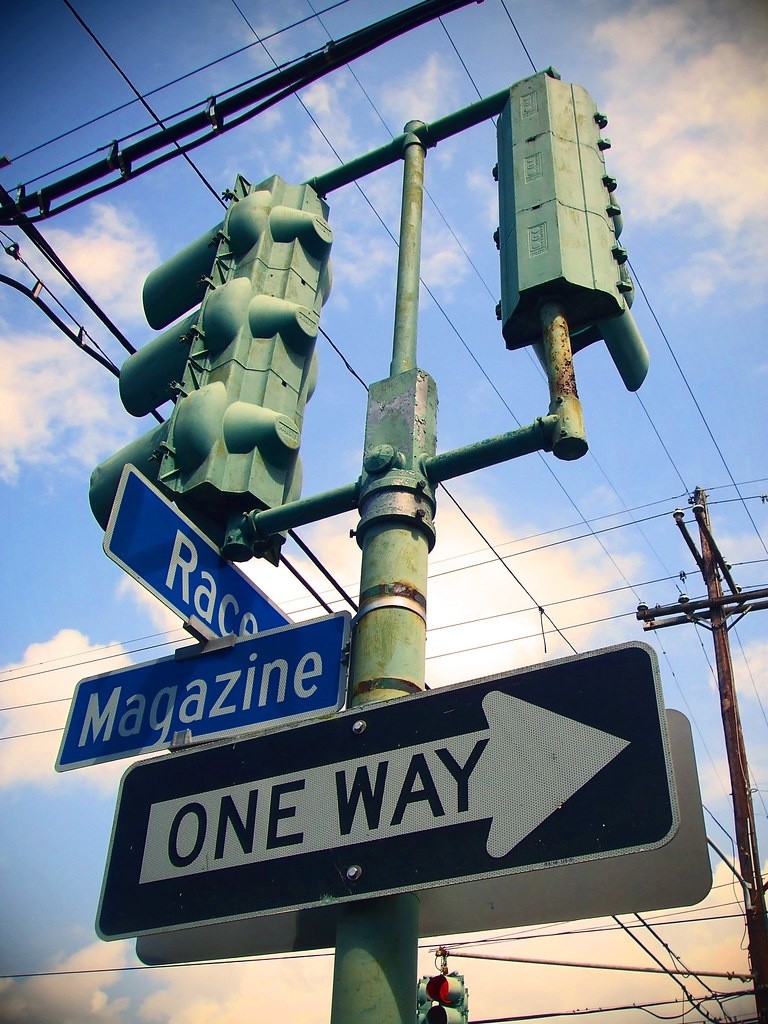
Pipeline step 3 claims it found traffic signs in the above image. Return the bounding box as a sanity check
[90,643,688,941]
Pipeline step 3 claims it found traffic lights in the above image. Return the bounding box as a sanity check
[87,170,333,565]
[414,952,477,1024]
